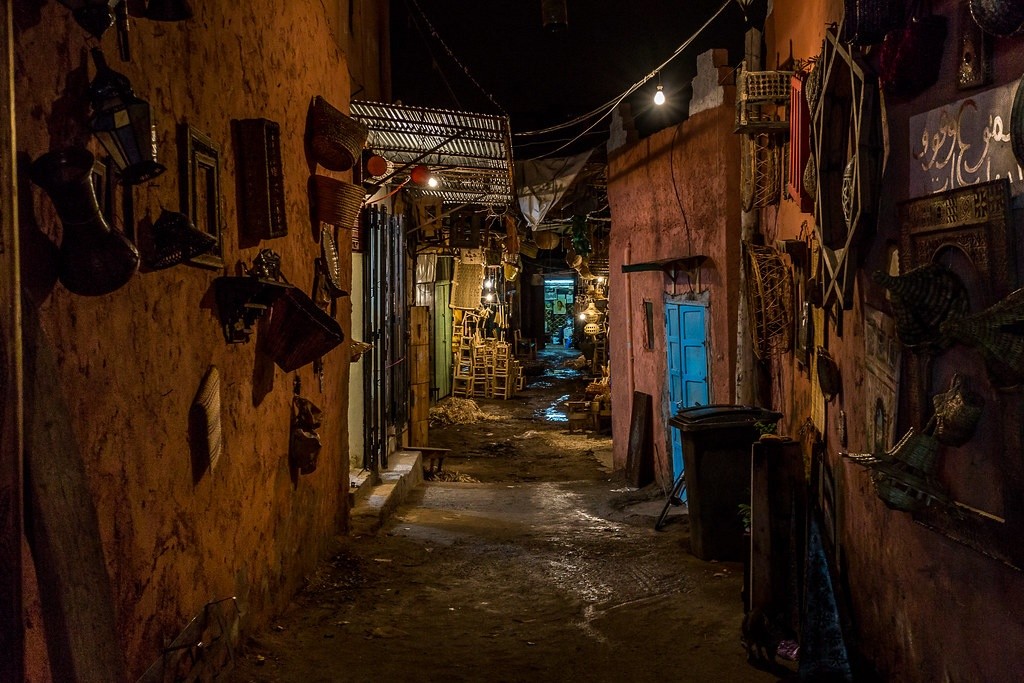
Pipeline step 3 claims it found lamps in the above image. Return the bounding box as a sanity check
[84,33,165,185]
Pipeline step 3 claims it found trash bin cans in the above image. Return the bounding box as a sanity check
[668,401,784,561]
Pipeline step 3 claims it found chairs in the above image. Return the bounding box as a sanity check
[451,321,526,400]
[514,329,537,355]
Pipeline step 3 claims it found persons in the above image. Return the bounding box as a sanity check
[557,301,563,311]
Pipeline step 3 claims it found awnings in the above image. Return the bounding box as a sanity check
[352,96,517,238]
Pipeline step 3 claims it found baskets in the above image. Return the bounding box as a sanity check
[740,132,781,213]
[315,173,366,230]
[267,271,344,373]
[314,95,368,172]
[740,240,794,360]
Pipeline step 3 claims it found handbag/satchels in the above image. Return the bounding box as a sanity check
[932,371,985,442]
